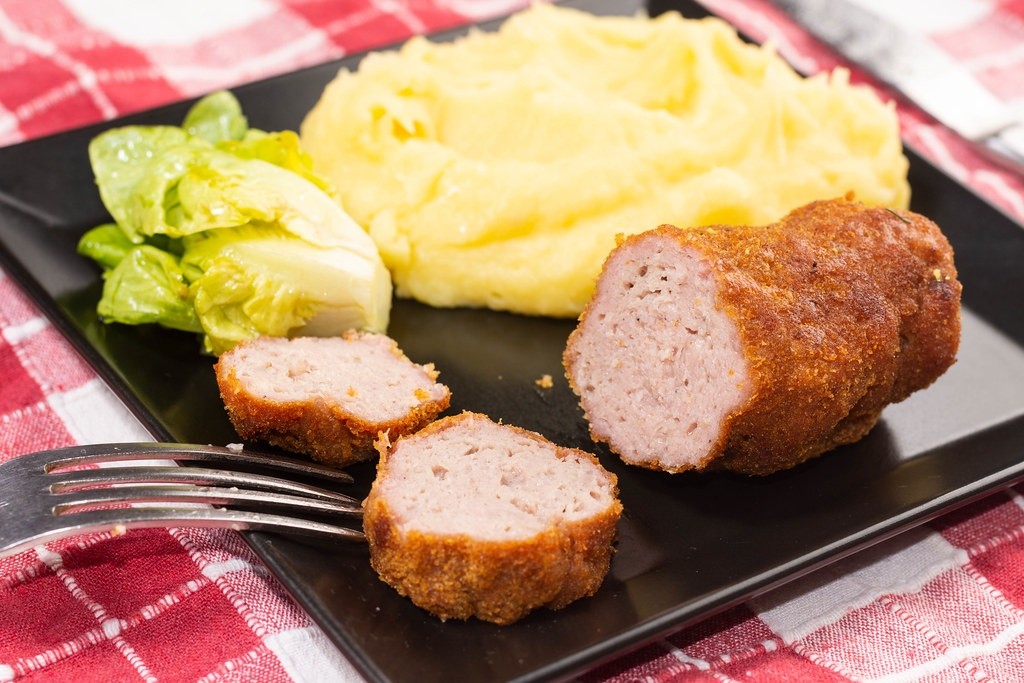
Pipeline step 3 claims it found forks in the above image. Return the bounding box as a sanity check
[0,441,373,566]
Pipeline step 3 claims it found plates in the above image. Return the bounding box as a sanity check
[0,1,1024,683]
